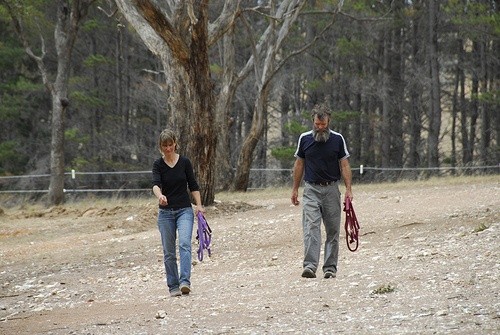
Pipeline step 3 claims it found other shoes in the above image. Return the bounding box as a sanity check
[302,267,316,278]
[323,270,336,279]
[169,284,190,296]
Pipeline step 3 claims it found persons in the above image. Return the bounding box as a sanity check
[151,129,205,297]
[291,102,353,278]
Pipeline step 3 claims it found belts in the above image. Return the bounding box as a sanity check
[305,180,337,186]
